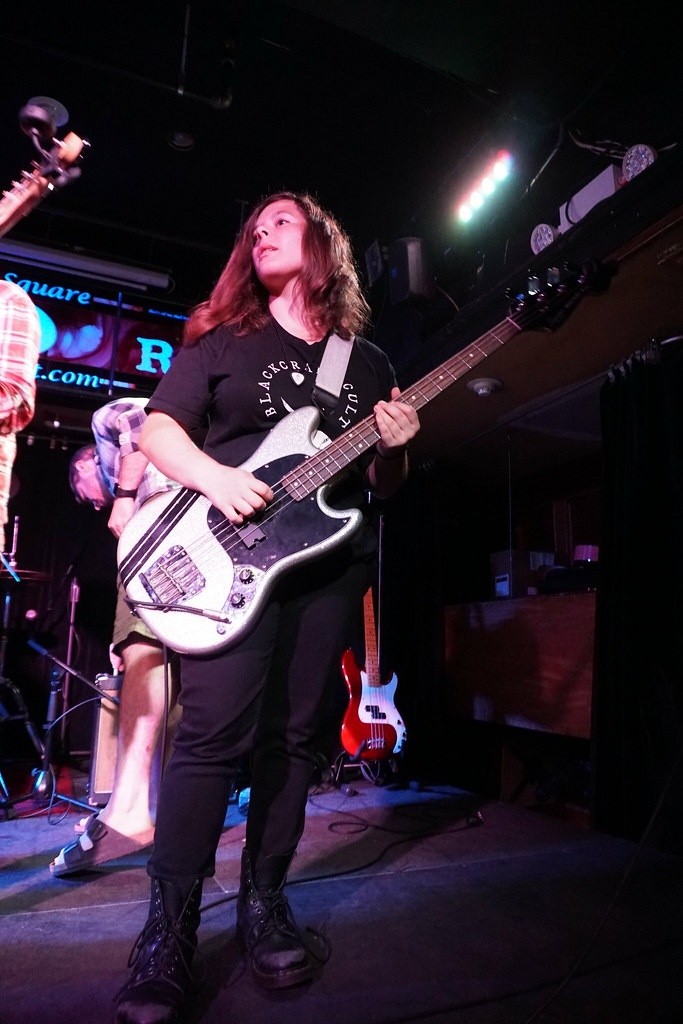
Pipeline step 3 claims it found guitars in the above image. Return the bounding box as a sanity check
[0,133,91,239]
[339,589,407,759]
[114,253,594,657]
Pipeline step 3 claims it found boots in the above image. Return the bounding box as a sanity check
[231,846,332,988]
[113,877,203,1024]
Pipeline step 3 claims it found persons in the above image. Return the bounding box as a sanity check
[0,280,41,554]
[48,397,184,877]
[114,191,420,1023]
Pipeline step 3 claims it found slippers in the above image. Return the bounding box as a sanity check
[74,809,102,834]
[50,818,156,875]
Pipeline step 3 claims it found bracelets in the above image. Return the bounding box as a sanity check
[113,483,138,499]
